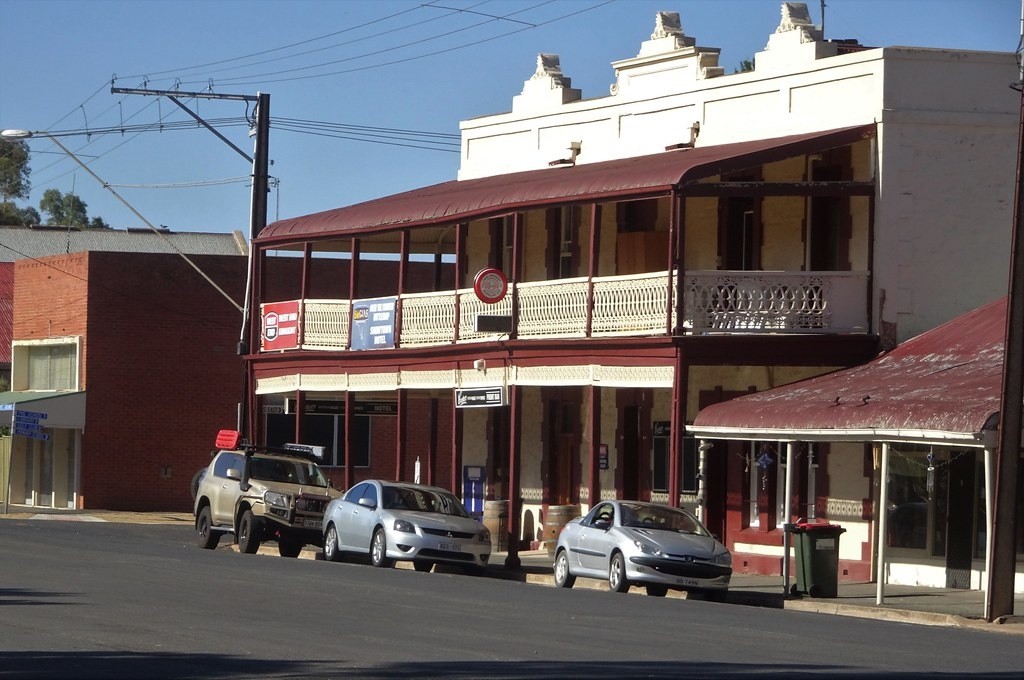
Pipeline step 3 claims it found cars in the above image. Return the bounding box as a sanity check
[554,500,732,601]
[322,480,492,574]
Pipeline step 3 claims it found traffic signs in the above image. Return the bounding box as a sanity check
[0,404,51,441]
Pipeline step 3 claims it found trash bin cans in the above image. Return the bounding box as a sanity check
[792,521,847,599]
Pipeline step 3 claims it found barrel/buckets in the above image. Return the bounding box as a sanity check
[482,500,520,552]
[545,506,580,559]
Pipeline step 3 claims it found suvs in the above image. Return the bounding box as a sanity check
[192,447,344,557]
